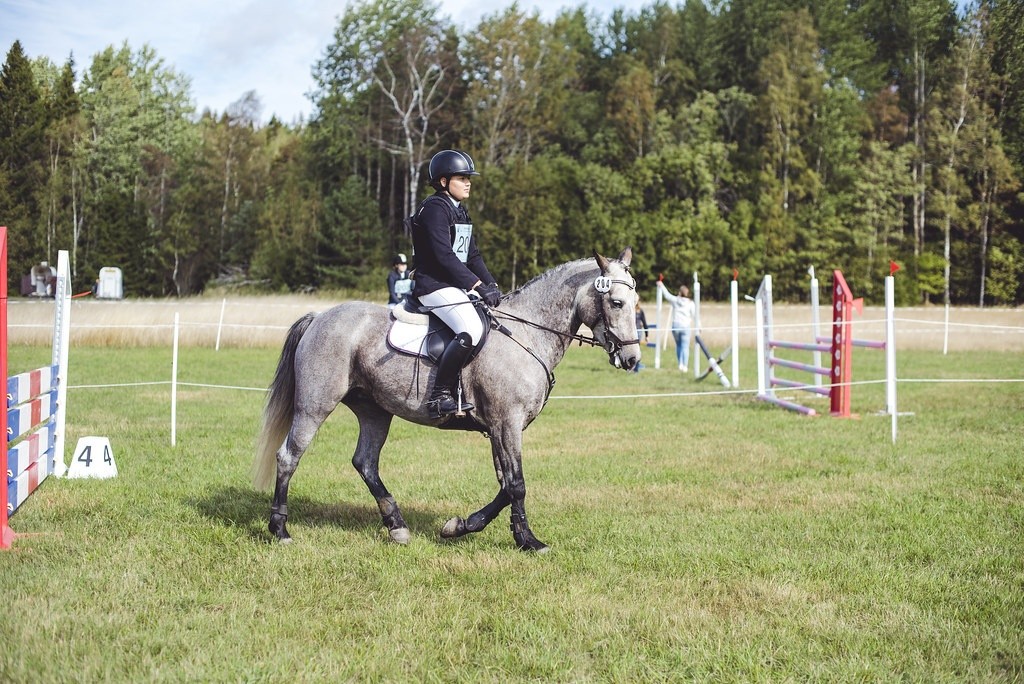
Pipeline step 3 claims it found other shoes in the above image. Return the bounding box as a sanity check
[678,364,688,373]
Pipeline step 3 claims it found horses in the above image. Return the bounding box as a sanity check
[250,249,643,556]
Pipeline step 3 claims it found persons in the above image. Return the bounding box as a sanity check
[657,281,695,372]
[403,150,501,419]
[387,254,412,309]
[627,302,649,374]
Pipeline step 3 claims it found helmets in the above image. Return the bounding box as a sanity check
[393,253,408,264]
[428,149,480,195]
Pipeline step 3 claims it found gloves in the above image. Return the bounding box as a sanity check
[474,282,501,307]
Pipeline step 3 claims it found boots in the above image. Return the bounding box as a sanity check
[427,332,474,420]
